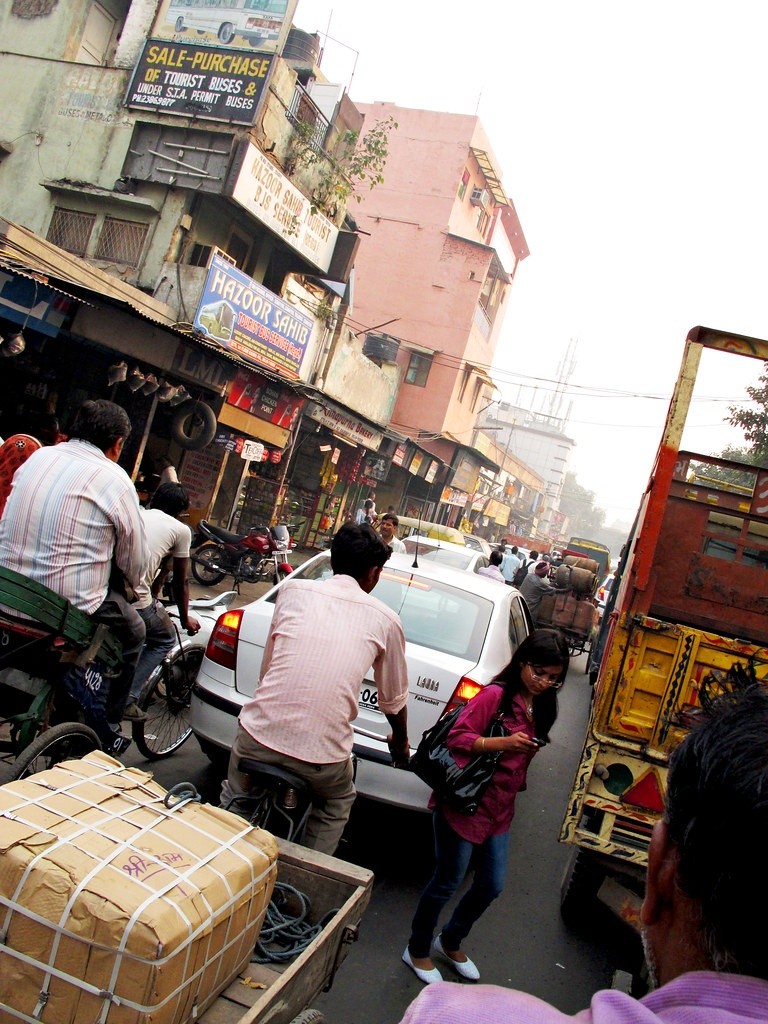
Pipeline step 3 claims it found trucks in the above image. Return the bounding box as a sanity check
[555,325,767,914]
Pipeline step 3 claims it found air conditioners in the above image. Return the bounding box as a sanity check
[470,187,490,209]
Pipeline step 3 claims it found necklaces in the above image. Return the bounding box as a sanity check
[523,696,533,717]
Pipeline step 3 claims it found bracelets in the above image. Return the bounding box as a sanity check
[482,738,486,752]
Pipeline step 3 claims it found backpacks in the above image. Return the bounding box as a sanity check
[513,559,535,586]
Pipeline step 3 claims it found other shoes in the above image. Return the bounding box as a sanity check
[122,703,149,723]
[434,932,481,980]
[112,725,133,741]
[402,944,443,984]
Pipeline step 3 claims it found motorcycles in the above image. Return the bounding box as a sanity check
[190,514,307,589]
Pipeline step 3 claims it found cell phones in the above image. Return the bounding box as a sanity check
[527,737,546,750]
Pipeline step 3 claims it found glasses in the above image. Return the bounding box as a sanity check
[527,662,562,689]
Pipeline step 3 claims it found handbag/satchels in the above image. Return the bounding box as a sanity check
[408,681,511,816]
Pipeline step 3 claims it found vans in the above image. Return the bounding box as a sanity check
[564,537,612,585]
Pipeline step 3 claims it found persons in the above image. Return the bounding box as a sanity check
[401,681,768,1023]
[0,399,152,731]
[501,539,508,546]
[528,552,557,585]
[220,523,409,857]
[153,455,179,496]
[122,481,200,720]
[500,546,523,584]
[379,513,406,554]
[520,551,539,573]
[355,491,394,526]
[401,628,568,984]
[519,562,575,614]
[477,551,505,584]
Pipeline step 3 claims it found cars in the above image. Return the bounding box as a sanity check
[593,573,616,621]
[188,512,552,817]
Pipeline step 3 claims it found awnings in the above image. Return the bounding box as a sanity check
[0,259,458,472]
[469,147,511,208]
[468,364,497,389]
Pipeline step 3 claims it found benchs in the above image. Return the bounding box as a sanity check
[0,566,124,725]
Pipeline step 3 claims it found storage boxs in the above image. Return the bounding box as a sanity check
[0,749,280,1024]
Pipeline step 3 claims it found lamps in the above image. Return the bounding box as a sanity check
[0,280,37,357]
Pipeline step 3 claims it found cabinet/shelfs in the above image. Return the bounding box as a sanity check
[236,474,342,549]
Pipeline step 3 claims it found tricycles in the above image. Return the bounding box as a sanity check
[0,718,376,1023]
[0,566,206,785]
